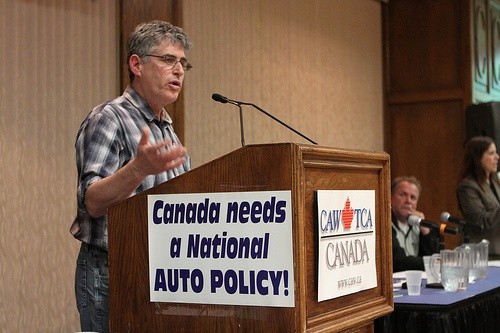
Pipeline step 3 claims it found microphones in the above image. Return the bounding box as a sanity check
[440,212,485,230]
[407,215,458,235]
[212,94,318,144]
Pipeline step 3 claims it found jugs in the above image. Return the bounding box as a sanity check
[429,243,489,292]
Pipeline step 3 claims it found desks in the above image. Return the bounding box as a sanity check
[373,257,500,333]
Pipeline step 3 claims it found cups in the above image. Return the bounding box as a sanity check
[405,271,422,296]
[423,256,440,284]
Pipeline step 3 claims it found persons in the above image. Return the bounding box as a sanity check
[456,135,500,255]
[391,175,440,273]
[70,20,192,333]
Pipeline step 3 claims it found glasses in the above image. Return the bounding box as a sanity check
[143,51,194,71]
